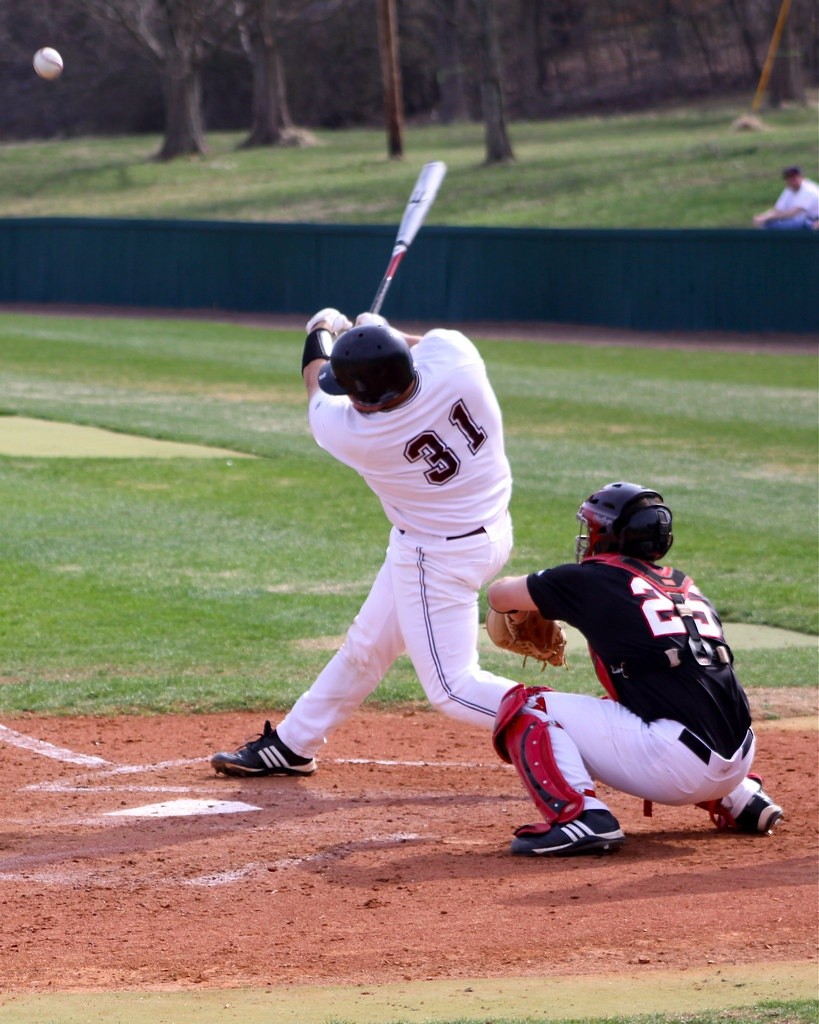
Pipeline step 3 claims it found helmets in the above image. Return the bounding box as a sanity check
[317,326,415,406]
[574,482,673,564]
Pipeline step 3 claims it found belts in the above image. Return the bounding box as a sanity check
[399,526,485,540]
[678,727,753,765]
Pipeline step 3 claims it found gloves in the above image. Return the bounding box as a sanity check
[306,308,353,335]
[356,313,400,340]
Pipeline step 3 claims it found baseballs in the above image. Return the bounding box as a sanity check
[32,47,66,78]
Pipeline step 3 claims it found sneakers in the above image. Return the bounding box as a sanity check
[509,809,626,855]
[211,720,316,777]
[734,789,784,836]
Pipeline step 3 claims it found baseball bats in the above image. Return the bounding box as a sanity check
[364,161,450,314]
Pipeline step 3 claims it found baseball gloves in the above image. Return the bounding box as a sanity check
[483,602,570,675]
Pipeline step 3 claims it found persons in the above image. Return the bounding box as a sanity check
[486,480,779,854]
[751,165,819,232]
[209,303,526,778]
